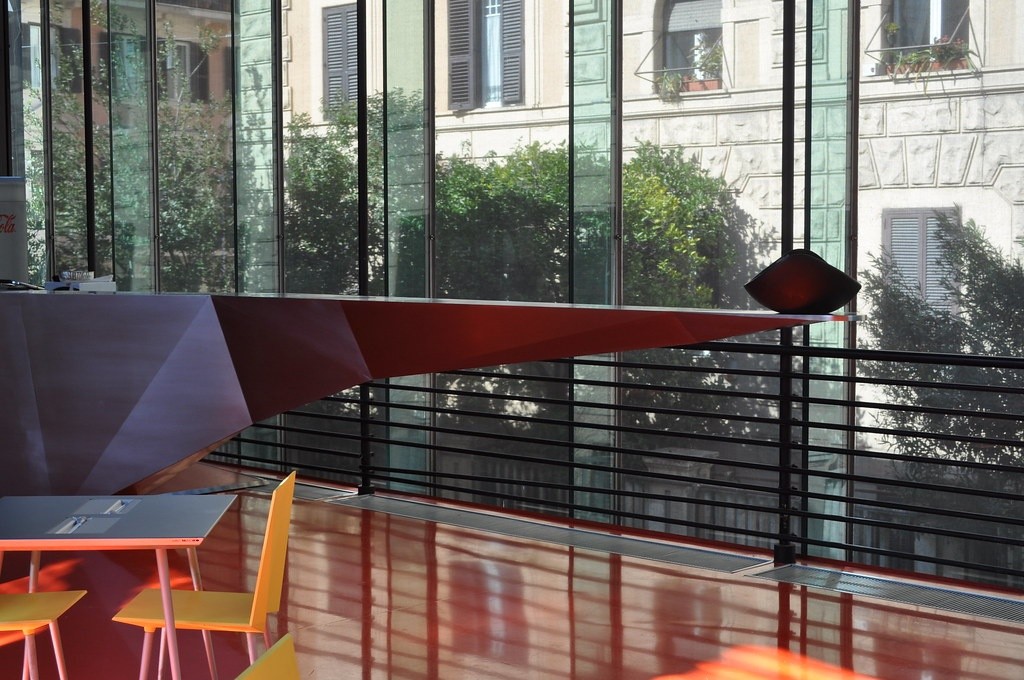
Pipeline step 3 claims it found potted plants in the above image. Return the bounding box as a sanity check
[881,22,982,89]
[652,38,722,103]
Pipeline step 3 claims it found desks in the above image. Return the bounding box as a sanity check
[0,495,236,680]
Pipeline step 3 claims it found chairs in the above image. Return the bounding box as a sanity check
[111,471,300,680]
[0,589,87,680]
